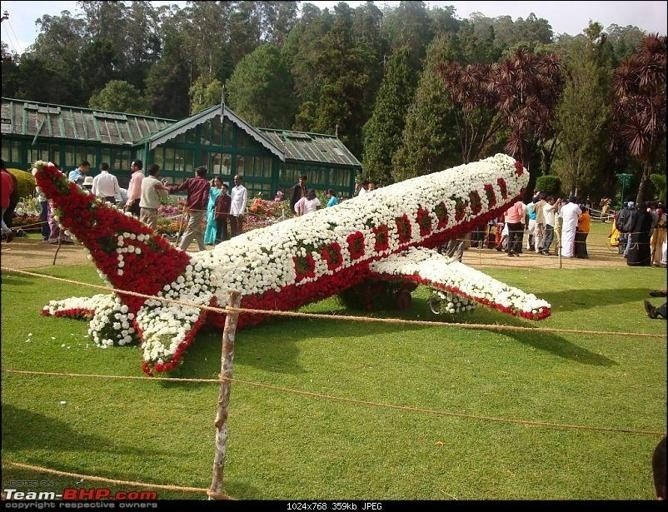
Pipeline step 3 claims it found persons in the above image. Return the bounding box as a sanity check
[644,290,668,319]
[438,190,668,267]
[1,159,383,253]
[652,438,667,501]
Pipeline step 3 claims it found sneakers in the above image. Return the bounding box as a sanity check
[5,229,14,242]
[471,238,550,257]
[643,299,659,319]
